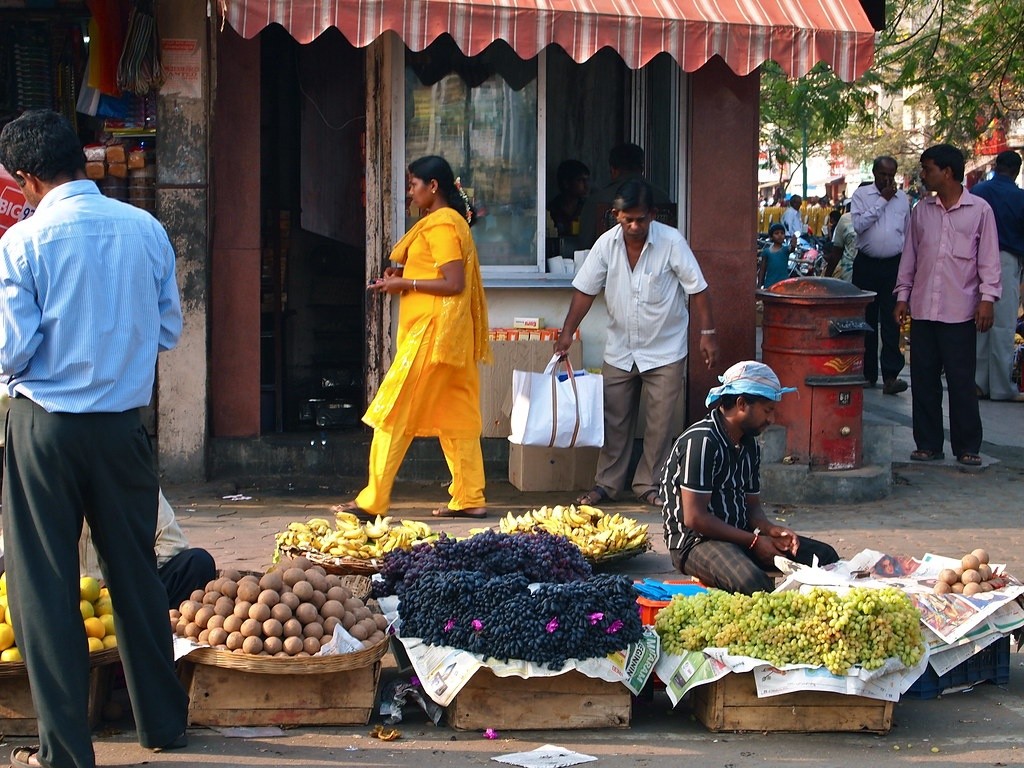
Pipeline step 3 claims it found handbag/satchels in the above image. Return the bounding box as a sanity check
[508,350,605,449]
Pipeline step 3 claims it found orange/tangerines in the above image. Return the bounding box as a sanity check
[0,571,116,664]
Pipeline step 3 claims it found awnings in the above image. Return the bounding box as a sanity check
[218,0,876,83]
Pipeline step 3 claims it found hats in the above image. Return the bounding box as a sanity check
[704,360,797,408]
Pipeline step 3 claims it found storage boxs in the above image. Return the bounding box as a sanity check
[509,441,600,493]
[189,663,380,727]
[0,666,99,735]
[902,634,1011,699]
[446,658,632,731]
[692,667,895,734]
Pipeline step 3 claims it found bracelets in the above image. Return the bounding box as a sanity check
[413,279,416,292]
[749,534,758,549]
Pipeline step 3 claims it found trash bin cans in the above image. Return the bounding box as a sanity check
[752,278,880,471]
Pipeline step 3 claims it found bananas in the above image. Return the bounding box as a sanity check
[274,511,491,561]
[499,504,651,559]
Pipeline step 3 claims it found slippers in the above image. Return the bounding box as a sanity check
[330,500,386,523]
[432,505,489,518]
[637,489,668,507]
[572,484,621,505]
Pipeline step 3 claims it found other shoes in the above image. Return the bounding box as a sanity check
[883,378,908,394]
[1010,392,1024,402]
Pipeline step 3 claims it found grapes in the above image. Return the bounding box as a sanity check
[369,527,645,671]
[654,585,926,675]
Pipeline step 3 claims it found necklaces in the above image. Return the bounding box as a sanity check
[724,429,741,449]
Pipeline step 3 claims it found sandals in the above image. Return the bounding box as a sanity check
[956,452,982,465]
[11,743,63,768]
[157,725,189,750]
[910,449,946,462]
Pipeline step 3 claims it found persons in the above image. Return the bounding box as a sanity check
[756,155,911,394]
[891,144,1002,466]
[553,172,721,507]
[968,150,1024,402]
[548,143,671,240]
[0,383,215,708]
[0,106,190,768]
[658,360,840,597]
[330,155,492,524]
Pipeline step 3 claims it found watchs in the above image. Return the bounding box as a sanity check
[700,328,720,336]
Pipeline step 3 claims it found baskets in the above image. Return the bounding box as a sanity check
[337,573,373,603]
